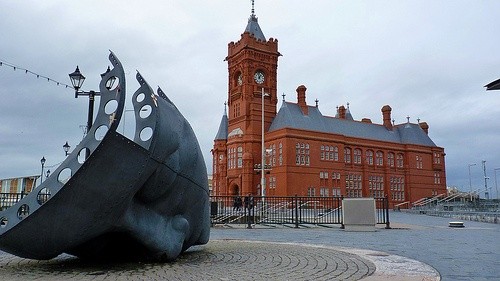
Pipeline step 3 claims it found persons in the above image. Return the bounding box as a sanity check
[233,192,254,211]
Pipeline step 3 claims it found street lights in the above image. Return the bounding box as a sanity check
[494,167,500,198]
[45,170,50,202]
[40,156,46,183]
[123,108,148,137]
[468,163,477,201]
[481,159,490,199]
[63,141,82,177]
[68,66,118,161]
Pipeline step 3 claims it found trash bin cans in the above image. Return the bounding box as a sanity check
[209,201,217,215]
